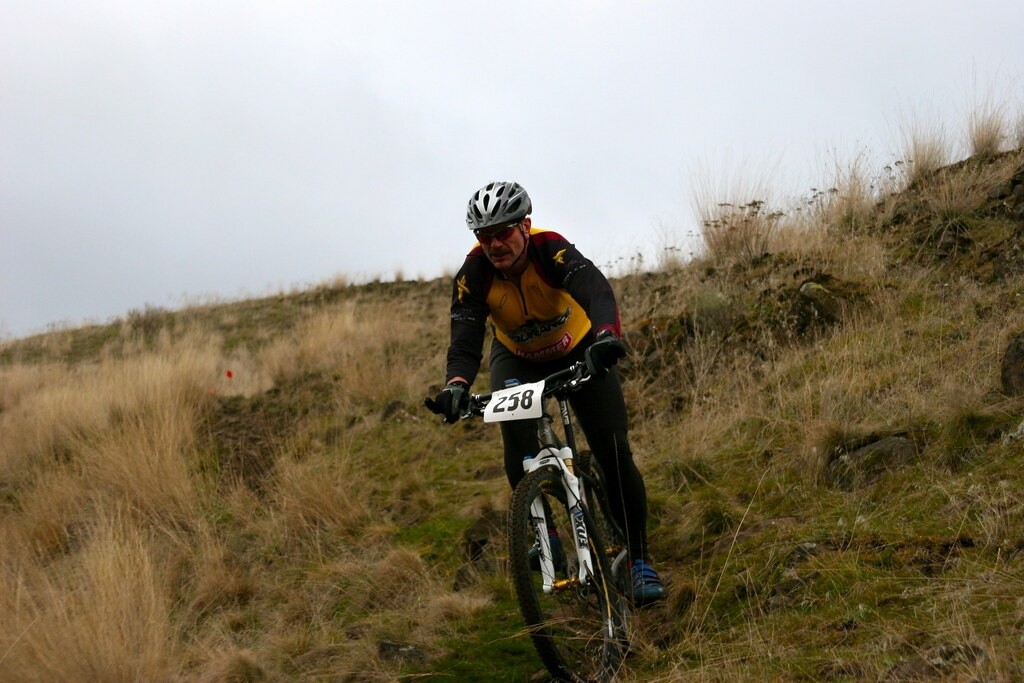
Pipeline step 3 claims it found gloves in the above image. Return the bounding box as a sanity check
[425,381,470,424]
[584,330,627,379]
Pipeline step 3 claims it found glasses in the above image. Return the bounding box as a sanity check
[473,221,522,244]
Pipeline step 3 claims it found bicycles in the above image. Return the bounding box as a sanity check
[425,360,630,683]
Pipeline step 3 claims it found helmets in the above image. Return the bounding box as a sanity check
[466,181,532,230]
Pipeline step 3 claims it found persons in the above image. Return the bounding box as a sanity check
[434,181,665,601]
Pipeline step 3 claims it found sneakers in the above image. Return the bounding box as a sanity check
[529,532,564,571]
[626,560,666,611]
